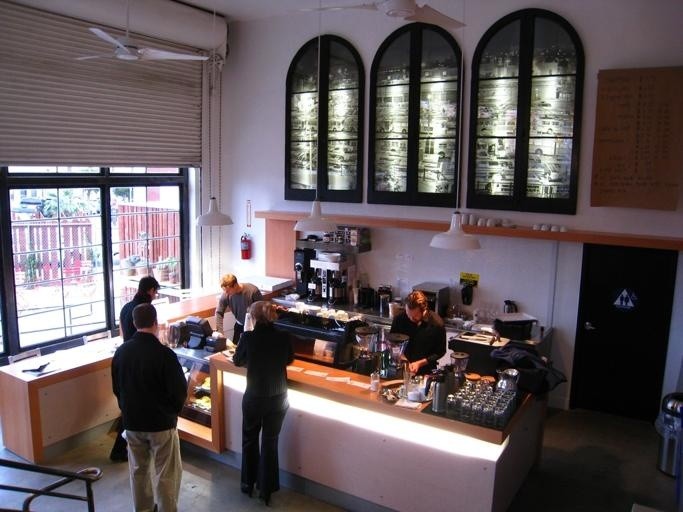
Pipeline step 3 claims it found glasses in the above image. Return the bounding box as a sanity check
[153,289,159,296]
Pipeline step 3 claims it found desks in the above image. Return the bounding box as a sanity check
[158,289,222,301]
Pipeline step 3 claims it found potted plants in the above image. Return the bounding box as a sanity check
[125,256,140,275]
[135,233,152,274]
[152,264,167,282]
[169,255,177,284]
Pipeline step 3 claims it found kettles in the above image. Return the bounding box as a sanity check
[424,369,449,413]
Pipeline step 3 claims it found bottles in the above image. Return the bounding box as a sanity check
[382,347,390,370]
[447,305,465,320]
[354,350,372,370]
[329,270,348,302]
[375,342,381,369]
[323,233,330,243]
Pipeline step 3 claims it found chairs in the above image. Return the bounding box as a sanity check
[8,348,41,364]
[180,289,197,302]
[151,297,169,307]
[83,330,111,345]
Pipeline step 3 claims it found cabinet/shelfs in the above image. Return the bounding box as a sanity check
[0,335,123,465]
[272,298,554,368]
[171,347,211,426]
[156,289,278,330]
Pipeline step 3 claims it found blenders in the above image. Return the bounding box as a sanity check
[449,350,471,389]
[352,324,382,376]
[378,332,410,380]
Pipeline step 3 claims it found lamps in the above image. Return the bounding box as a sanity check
[294,0,339,232]
[429,0,481,250]
[195,0,233,226]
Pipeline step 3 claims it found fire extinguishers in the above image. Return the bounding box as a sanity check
[241,232,251,259]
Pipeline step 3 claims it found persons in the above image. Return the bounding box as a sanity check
[216,274,264,345]
[111,304,187,512]
[232,301,295,506]
[110,277,161,462]
[388,290,447,379]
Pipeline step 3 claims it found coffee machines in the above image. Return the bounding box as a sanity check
[305,249,356,305]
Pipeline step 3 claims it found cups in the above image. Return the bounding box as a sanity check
[445,381,517,429]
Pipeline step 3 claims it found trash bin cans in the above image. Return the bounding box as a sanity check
[654,390,683,478]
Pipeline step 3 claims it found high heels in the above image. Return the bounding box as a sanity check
[242,483,253,497]
[259,490,271,505]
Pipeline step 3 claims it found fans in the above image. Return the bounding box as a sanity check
[299,0,467,31]
[74,0,210,61]
